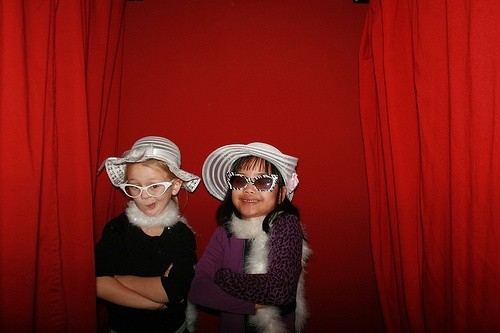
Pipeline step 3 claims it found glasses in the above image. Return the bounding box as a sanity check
[226,172,278,192]
[119,178,177,199]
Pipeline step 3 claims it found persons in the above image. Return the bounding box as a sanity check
[94,135,201,333]
[188,141,311,333]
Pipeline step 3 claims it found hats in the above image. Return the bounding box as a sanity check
[104,136,201,192]
[202,142,299,203]
